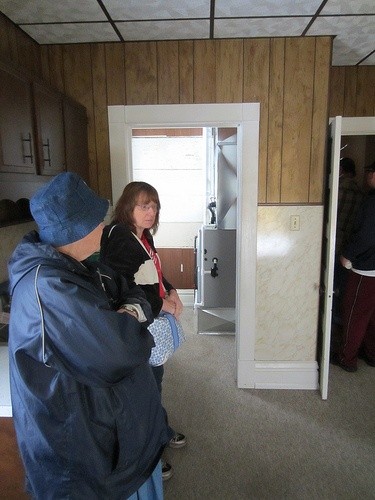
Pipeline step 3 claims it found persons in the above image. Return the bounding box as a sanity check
[329,156,375,373]
[98,181,187,480]
[7,172,182,500]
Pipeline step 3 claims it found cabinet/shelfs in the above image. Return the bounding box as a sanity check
[162,250,194,288]
[62,98,89,186]
[0,56,66,178]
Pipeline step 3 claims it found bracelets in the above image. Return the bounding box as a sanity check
[121,306,137,314]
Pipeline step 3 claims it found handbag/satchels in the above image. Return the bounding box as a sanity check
[147,313,186,366]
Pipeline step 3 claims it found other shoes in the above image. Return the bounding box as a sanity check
[330,354,357,372]
[358,351,375,367]
[169,433,187,448]
[161,463,174,480]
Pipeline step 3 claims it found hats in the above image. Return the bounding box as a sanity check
[29,171,109,248]
